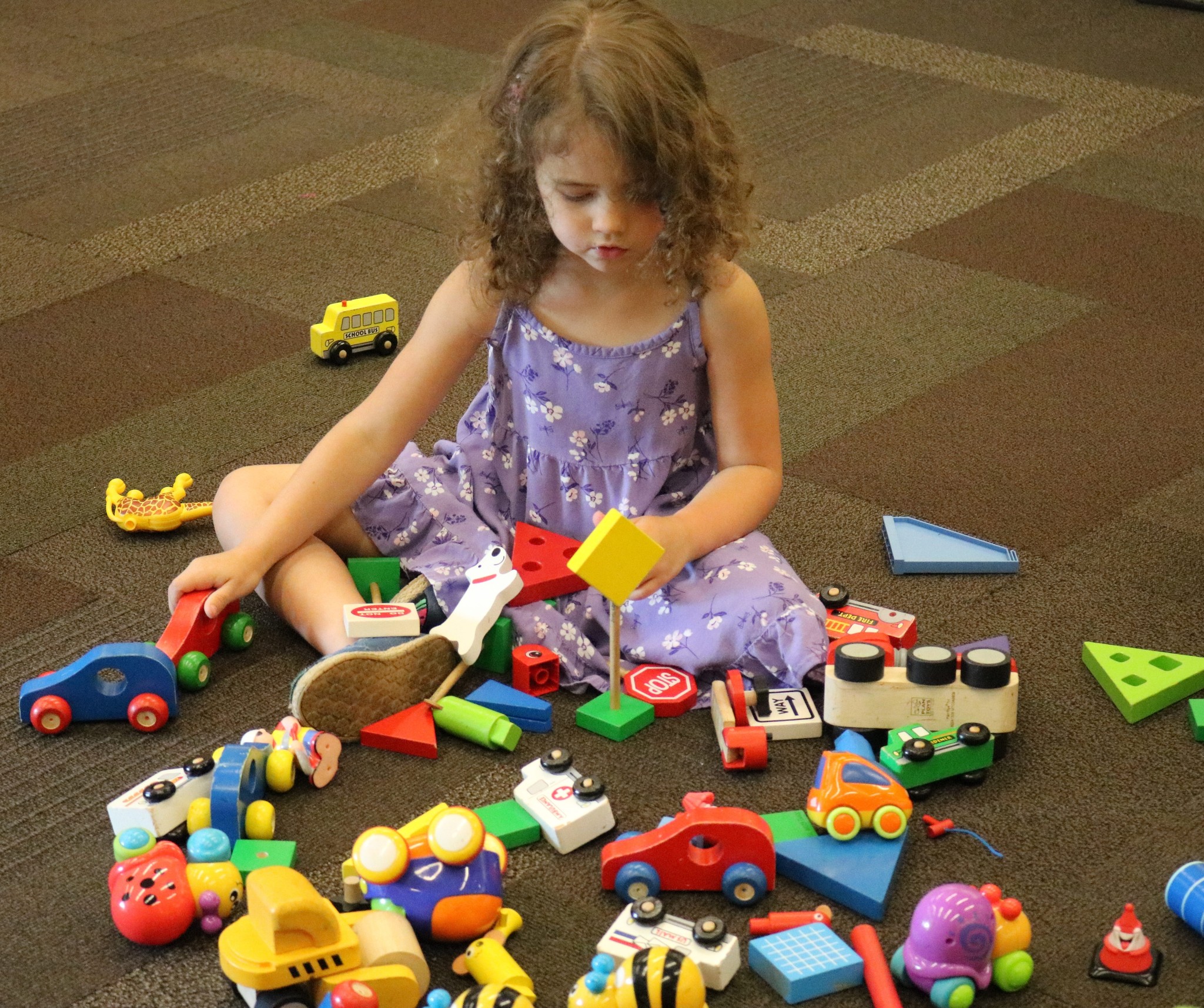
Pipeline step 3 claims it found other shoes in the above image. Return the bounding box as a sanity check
[390,572,447,634]
[289,635,461,743]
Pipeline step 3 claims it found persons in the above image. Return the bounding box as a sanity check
[168,1,828,744]
